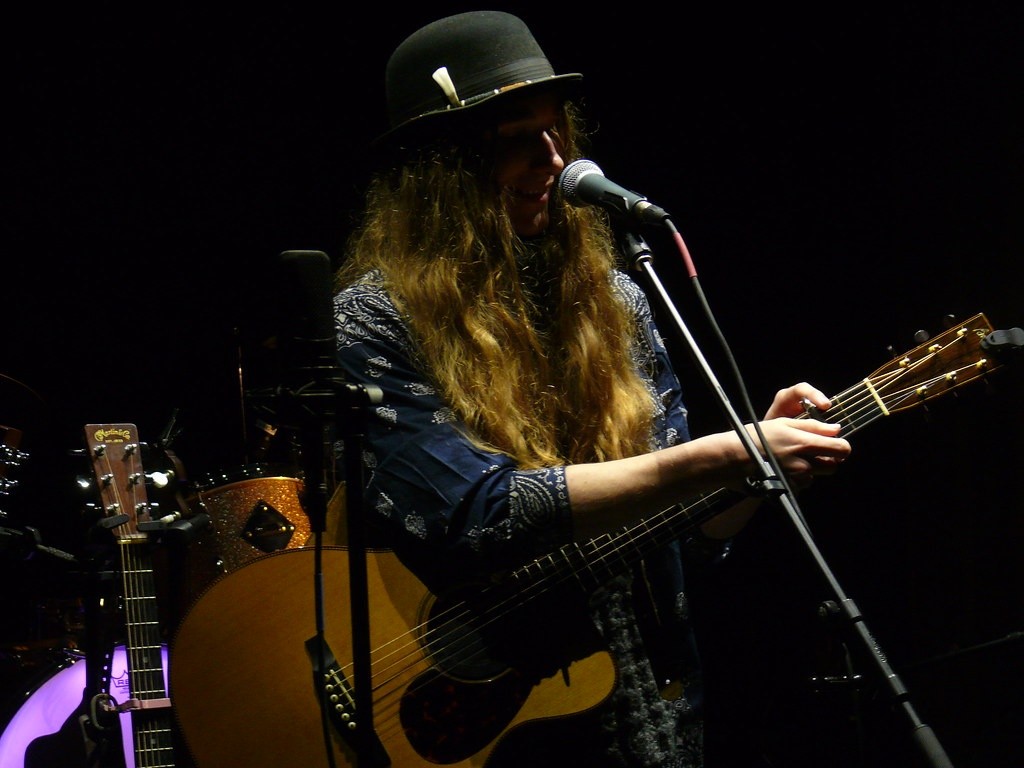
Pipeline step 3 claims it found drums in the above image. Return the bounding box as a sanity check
[0,634,183,768]
[176,468,350,594]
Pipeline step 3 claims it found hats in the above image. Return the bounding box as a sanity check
[370,10,585,152]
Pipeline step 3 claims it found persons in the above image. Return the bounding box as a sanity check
[332,10,852,768]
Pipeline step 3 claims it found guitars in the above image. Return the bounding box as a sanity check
[64,415,198,768]
[152,304,1019,768]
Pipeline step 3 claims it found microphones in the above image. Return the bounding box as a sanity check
[557,160,671,226]
[280,249,339,531]
